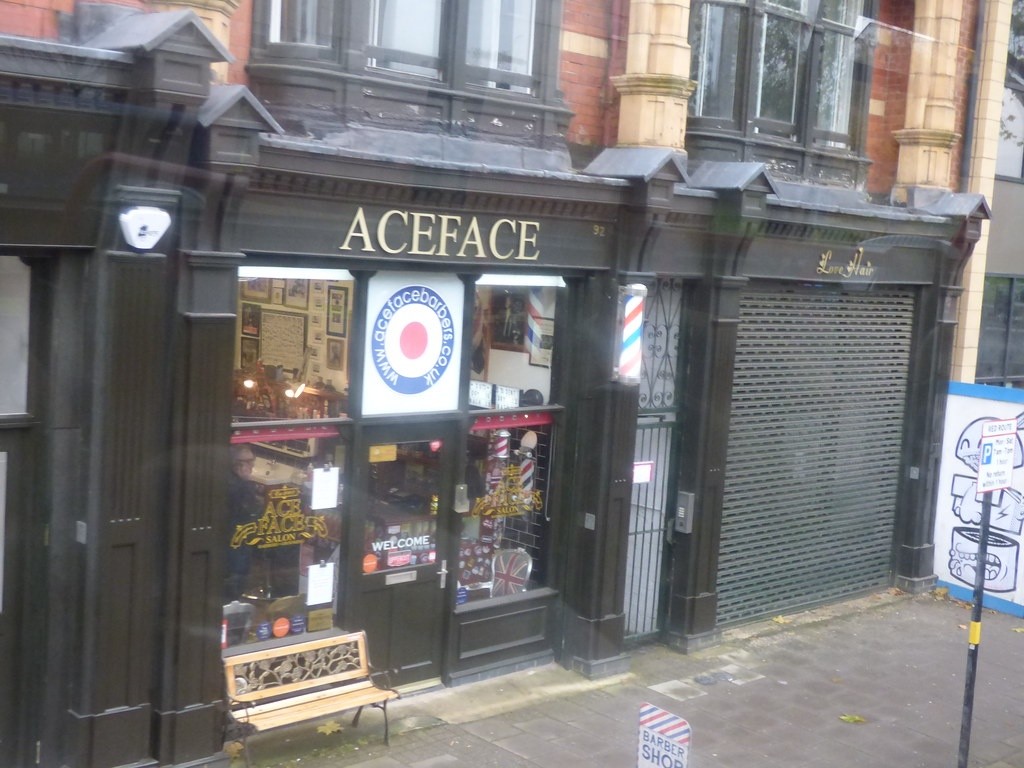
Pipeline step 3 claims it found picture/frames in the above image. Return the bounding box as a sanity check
[488,289,530,354]
[526,316,555,368]
[238,277,351,374]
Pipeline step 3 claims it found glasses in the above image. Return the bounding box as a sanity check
[238,456,257,465]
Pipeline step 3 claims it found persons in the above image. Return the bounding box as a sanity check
[223,444,264,604]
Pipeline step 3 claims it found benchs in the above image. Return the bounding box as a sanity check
[218,628,406,768]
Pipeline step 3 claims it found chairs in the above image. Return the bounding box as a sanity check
[488,546,534,602]
